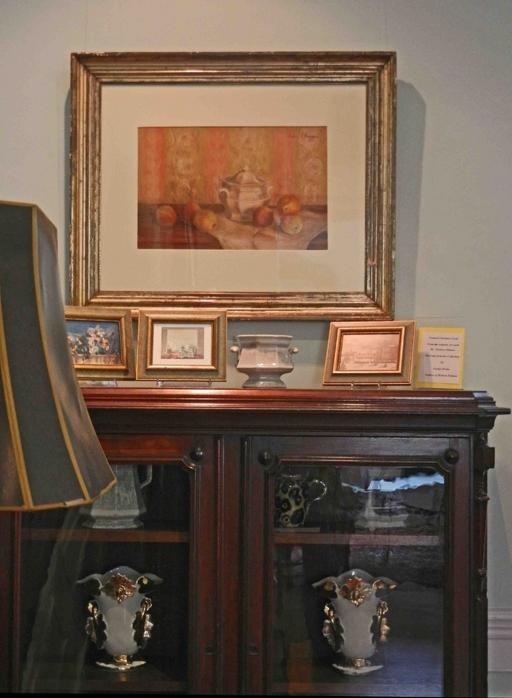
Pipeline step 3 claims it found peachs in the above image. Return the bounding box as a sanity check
[253,195,304,234]
[156,202,218,232]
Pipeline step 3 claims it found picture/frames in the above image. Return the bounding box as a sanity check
[324,316,416,384]
[67,48,399,322]
[58,303,224,385]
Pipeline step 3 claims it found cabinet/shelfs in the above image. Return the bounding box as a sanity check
[2,421,481,695]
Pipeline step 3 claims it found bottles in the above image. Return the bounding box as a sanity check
[79,463,153,530]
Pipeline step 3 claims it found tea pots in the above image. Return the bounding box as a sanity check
[277,473,327,528]
